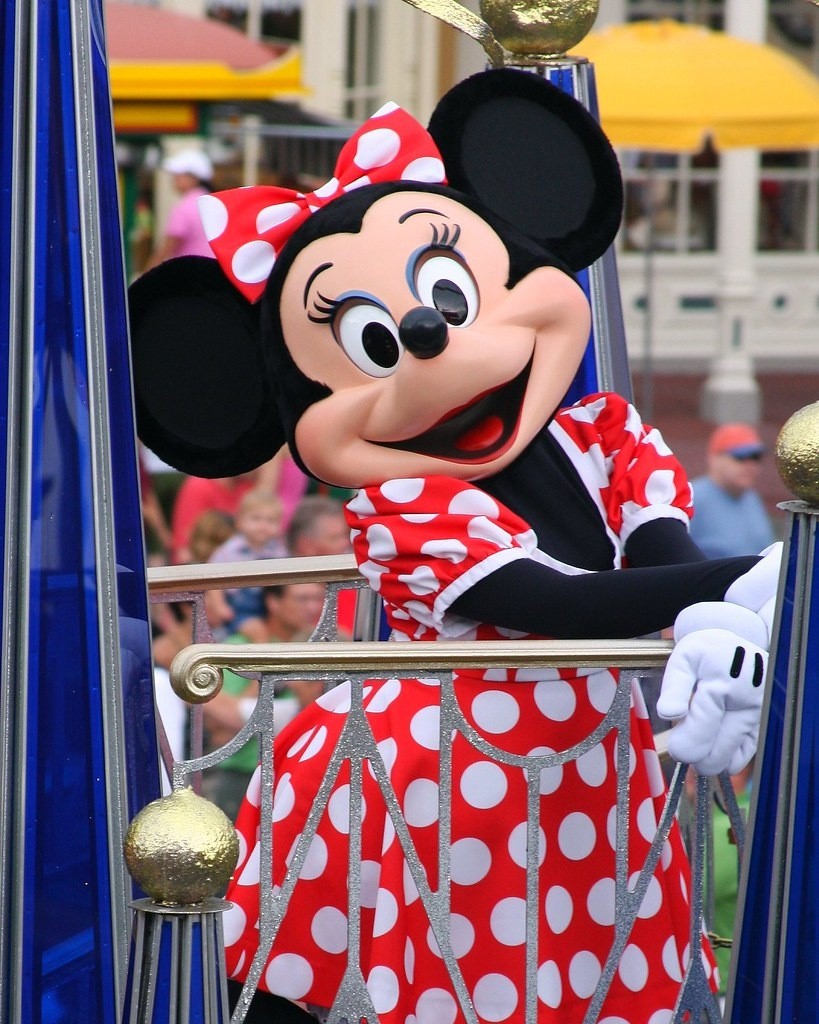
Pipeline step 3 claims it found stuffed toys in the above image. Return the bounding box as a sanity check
[126,66,784,1024]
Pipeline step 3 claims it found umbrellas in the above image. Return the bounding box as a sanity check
[564,20,819,424]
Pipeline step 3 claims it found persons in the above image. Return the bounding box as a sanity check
[143,150,221,271]
[660,423,777,802]
[139,446,356,800]
